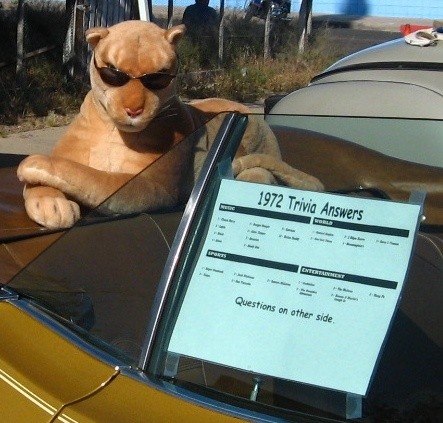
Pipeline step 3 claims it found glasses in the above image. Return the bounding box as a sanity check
[94,49,179,89]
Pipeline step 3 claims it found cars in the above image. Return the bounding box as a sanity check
[263,34,443,166]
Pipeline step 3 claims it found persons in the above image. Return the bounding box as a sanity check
[182,0,217,33]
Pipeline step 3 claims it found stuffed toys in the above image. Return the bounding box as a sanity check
[16,18,325,232]
